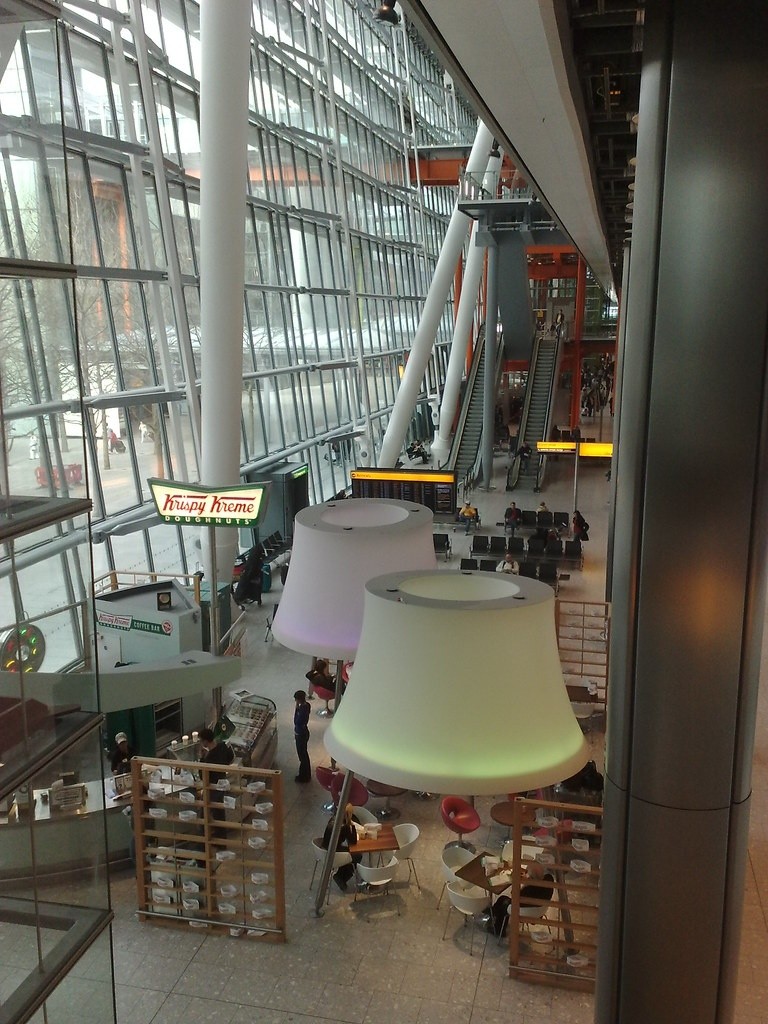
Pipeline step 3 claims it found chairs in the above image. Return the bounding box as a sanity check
[233,529,293,573]
[551,430,595,454]
[432,507,582,594]
[311,760,604,959]
[325,491,349,503]
[312,683,334,719]
[395,440,433,468]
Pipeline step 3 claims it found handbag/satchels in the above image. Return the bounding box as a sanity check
[578,517,589,533]
[580,532,589,541]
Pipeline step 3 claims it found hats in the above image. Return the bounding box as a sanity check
[465,501,471,505]
[115,731,127,745]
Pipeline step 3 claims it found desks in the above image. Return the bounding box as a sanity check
[349,824,400,895]
[490,801,536,845]
[453,851,529,933]
[365,779,408,821]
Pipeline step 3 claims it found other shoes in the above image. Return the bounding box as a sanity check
[481,908,495,917]
[486,925,506,938]
[333,873,348,891]
[464,531,468,536]
[193,860,206,868]
[294,775,310,783]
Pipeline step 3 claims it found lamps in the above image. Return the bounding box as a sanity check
[324,567,594,795]
[273,495,436,663]
[372,0,400,27]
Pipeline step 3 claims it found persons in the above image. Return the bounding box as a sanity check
[527,528,557,565]
[305,665,346,695]
[459,501,476,536]
[199,729,234,850]
[581,354,615,416]
[106,423,118,453]
[536,501,549,513]
[555,310,565,338]
[111,732,137,776]
[407,441,431,464]
[139,421,147,443]
[29,432,38,459]
[573,511,584,542]
[323,804,362,892]
[294,691,311,782]
[483,862,555,929]
[514,442,532,472]
[496,554,519,575]
[506,502,519,536]
